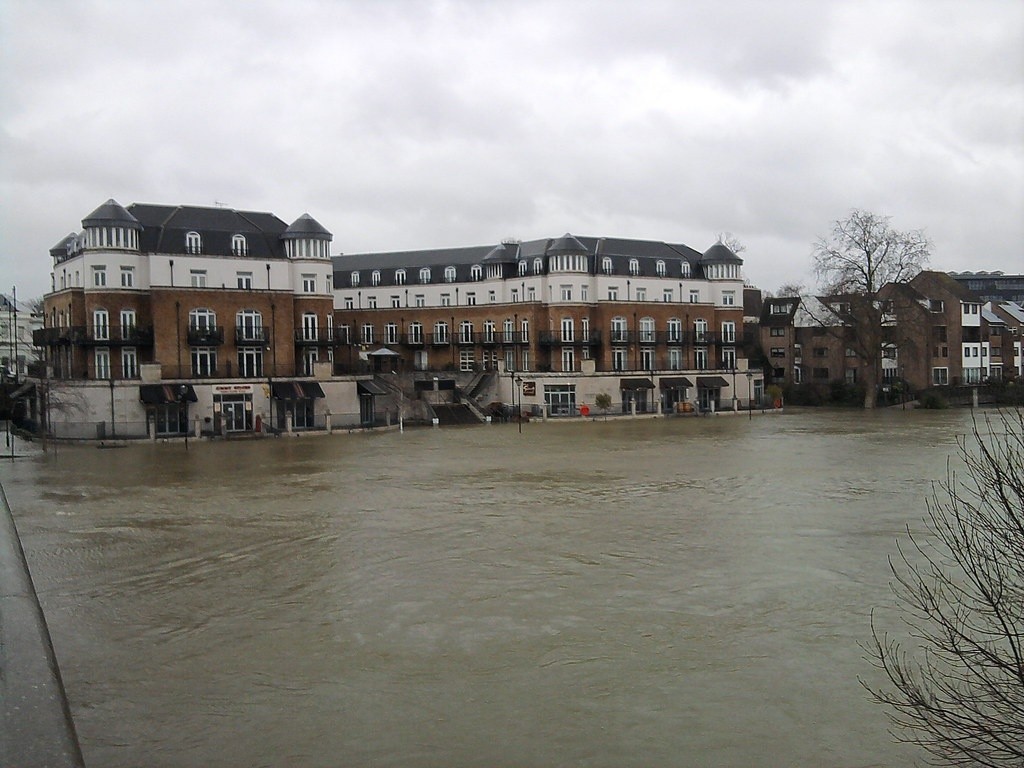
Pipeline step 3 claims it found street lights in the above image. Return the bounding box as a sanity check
[746,372,754,420]
[180,384,189,450]
[515,375,523,434]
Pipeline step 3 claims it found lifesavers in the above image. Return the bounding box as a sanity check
[581,406,589,416]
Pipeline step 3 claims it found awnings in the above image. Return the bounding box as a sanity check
[9,383,37,399]
[358,381,387,396]
[659,377,694,389]
[620,379,656,390]
[271,382,325,399]
[697,377,729,387]
[139,385,198,404]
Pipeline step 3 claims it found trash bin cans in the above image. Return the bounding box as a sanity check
[95,420,106,440]
[531,404,540,416]
[512,406,521,416]
